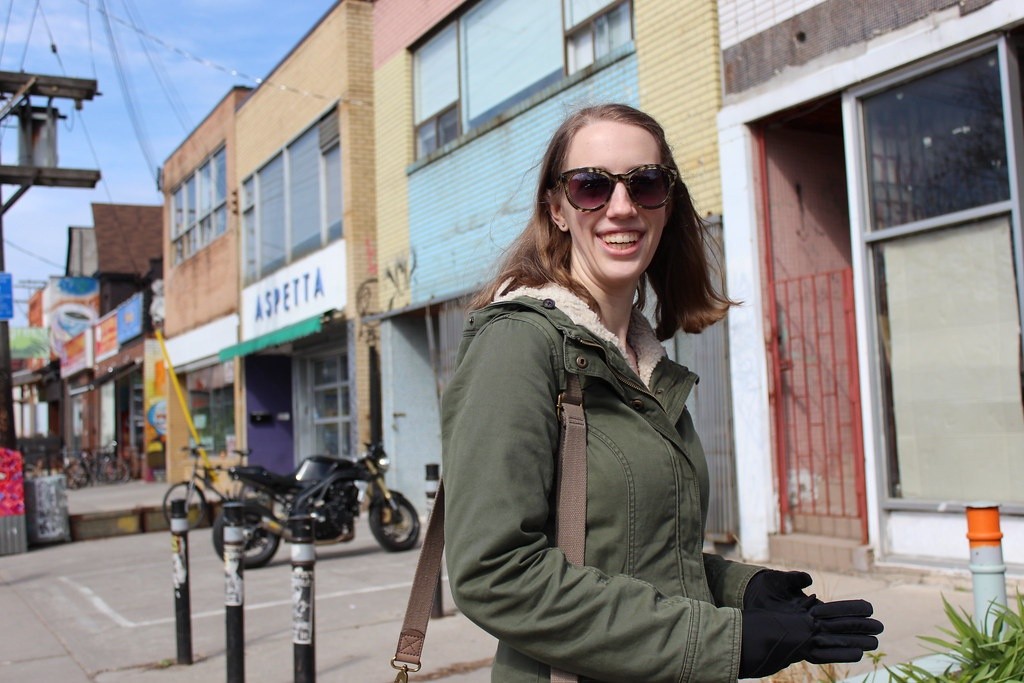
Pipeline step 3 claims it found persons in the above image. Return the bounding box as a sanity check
[30,456,43,480]
[438,104,885,683]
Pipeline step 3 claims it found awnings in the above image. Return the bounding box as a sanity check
[69,359,136,397]
[219,308,334,364]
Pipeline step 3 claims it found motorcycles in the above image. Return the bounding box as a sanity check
[213,435,421,570]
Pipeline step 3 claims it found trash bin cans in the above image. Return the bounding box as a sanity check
[24,475,70,543]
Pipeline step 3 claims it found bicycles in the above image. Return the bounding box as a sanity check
[160,441,254,530]
[54,439,132,491]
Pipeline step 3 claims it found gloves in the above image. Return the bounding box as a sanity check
[743,570,884,664]
[739,594,821,679]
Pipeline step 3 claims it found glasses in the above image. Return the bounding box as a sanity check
[552,164,678,210]
